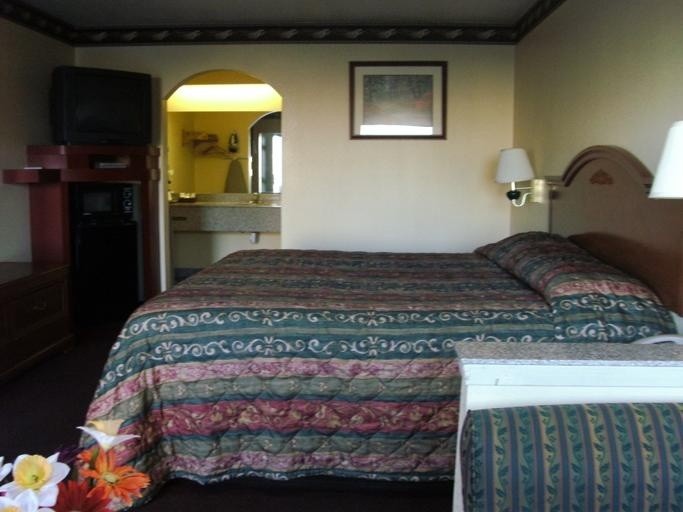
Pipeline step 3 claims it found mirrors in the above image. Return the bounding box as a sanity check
[165,69,283,202]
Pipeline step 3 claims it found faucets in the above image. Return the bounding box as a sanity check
[248,193,260,204]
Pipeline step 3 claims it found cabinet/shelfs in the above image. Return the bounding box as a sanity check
[0,258,72,387]
[2,143,150,334]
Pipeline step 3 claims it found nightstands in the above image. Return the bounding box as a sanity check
[451,339,683,512]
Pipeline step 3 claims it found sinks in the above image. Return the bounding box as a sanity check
[238,201,272,205]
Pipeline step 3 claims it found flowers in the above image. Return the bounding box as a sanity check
[0,417,152,512]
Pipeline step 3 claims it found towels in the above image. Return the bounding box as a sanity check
[224,159,248,193]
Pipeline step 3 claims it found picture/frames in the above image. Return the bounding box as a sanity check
[349,59,448,140]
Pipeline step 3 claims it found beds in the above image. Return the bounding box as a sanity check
[75,145,683,512]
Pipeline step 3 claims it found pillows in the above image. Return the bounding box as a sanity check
[460,401,683,512]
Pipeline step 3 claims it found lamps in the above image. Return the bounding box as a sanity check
[492,147,546,209]
[647,119,683,200]
